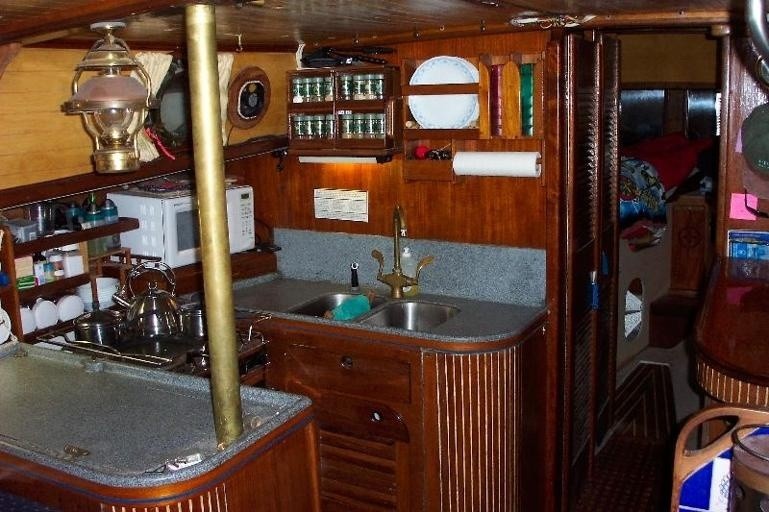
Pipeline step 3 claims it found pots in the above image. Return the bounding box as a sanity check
[179,296,217,345]
[73,301,160,344]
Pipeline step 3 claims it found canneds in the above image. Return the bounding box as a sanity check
[291,74,386,140]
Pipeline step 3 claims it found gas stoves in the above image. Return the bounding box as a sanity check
[37,324,207,368]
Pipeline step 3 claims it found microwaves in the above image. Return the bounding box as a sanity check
[104,180,255,270]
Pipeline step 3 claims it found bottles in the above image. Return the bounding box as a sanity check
[729,232,769,280]
[292,73,386,140]
[26,191,121,286]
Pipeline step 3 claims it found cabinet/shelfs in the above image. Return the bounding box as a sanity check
[287,66,401,157]
[267,319,424,512]
[401,50,547,186]
[0,135,288,344]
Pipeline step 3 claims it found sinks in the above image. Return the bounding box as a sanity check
[362,299,461,332]
[287,292,389,323]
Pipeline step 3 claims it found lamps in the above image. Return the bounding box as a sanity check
[298,156,386,163]
[60,21,160,173]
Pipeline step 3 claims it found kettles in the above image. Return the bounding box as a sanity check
[112,260,185,340]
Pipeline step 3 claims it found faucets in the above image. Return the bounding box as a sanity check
[392,204,408,274]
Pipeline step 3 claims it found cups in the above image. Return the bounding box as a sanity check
[728,425,768,511]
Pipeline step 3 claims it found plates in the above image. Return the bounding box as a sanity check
[407,55,480,129]
[19,277,120,334]
